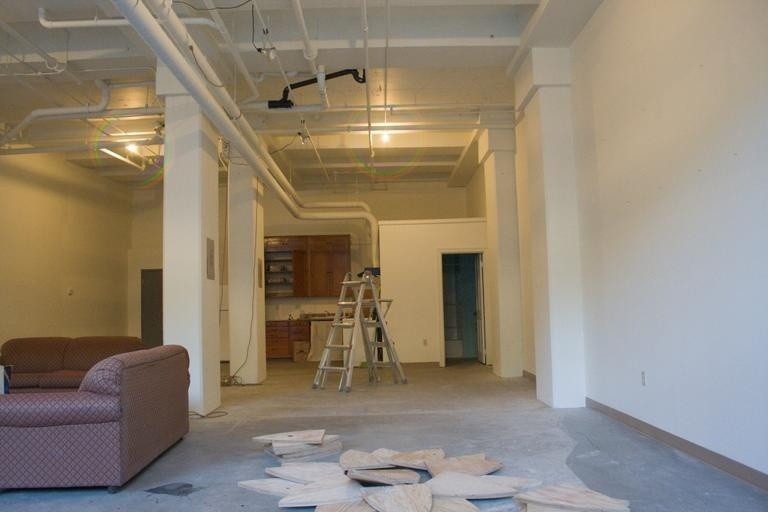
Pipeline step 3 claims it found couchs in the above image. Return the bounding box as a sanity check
[0,335,190,493]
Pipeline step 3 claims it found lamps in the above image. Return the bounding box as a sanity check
[298,132,310,145]
[257,48,277,60]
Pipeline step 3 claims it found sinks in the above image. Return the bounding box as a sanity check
[312,316,350,319]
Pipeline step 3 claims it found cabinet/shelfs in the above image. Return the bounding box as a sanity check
[265,320,310,358]
[263,234,352,298]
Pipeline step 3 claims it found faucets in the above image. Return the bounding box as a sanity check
[324,310,331,316]
